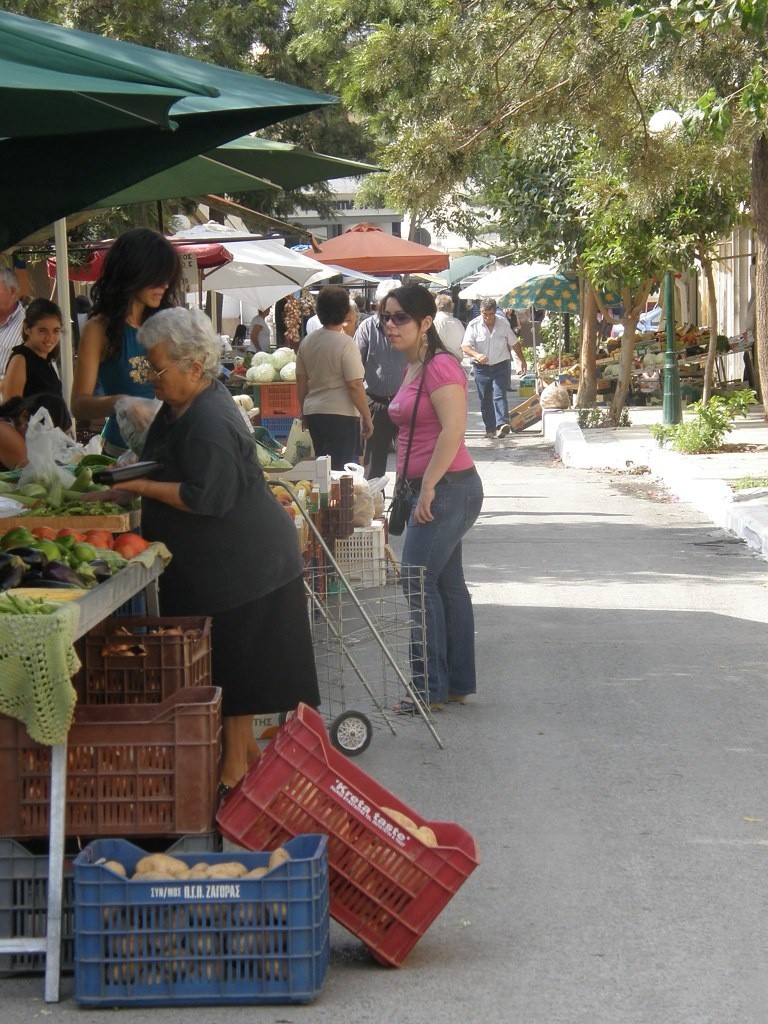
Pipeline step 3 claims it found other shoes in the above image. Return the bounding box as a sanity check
[485,431,496,438]
[497,424,511,438]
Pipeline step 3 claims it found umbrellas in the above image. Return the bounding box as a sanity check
[299,222,449,273]
[174,220,339,311]
[40,238,232,283]
[459,264,623,381]
[0,11,393,255]
[429,256,495,293]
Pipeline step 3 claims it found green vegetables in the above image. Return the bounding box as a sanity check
[707,335,731,355]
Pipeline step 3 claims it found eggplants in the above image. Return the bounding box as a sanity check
[0,546,114,591]
[685,345,703,357]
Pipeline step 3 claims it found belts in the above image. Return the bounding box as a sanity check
[408,465,477,489]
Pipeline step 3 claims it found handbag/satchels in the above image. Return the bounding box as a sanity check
[114,398,165,459]
[16,406,79,489]
[389,479,408,537]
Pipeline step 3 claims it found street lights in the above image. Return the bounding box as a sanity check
[644,110,690,433]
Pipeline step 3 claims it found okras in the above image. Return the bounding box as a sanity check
[14,499,128,517]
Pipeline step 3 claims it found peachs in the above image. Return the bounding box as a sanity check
[263,471,312,519]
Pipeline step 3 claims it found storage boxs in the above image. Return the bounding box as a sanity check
[301,542,331,618]
[261,417,295,440]
[70,615,213,705]
[71,834,330,1005]
[212,703,482,970]
[321,474,354,541]
[0,686,224,836]
[508,395,543,433]
[537,325,754,405]
[268,455,332,508]
[333,520,386,590]
[0,832,215,979]
[260,385,302,417]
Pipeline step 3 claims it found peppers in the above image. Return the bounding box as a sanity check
[0,591,57,616]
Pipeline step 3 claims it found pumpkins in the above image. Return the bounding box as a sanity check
[232,394,253,411]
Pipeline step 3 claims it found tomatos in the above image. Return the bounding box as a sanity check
[32,526,149,558]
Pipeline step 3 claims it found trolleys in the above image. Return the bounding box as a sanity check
[264,478,448,758]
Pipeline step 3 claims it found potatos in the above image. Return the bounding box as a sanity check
[257,775,441,931]
[350,482,384,527]
[98,847,291,985]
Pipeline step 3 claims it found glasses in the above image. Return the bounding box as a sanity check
[380,313,413,326]
[142,356,194,382]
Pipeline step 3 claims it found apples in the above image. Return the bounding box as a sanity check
[537,358,569,370]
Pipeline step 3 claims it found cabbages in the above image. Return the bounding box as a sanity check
[246,347,298,383]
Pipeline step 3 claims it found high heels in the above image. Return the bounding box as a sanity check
[394,701,444,711]
[448,694,469,704]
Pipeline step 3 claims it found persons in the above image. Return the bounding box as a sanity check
[70,228,184,460]
[247,306,271,355]
[295,287,373,471]
[379,284,486,712]
[470,299,482,318]
[433,294,464,360]
[496,309,517,392]
[82,307,322,795]
[0,267,72,473]
[504,308,521,335]
[461,298,526,438]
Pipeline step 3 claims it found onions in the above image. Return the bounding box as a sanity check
[95,629,199,705]
